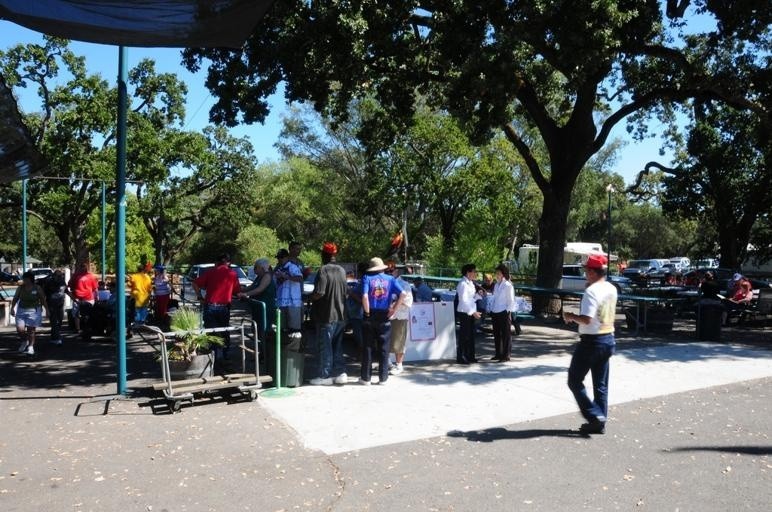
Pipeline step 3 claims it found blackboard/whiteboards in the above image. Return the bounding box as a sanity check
[390,301,457,363]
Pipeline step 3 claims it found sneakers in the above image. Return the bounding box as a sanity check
[359,378,371,385]
[309,378,333,385]
[581,416,606,432]
[330,373,348,384]
[19,342,27,352]
[27,346,35,354]
[375,362,394,371]
[56,340,62,345]
[388,365,403,375]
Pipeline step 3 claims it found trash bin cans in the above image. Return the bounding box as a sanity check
[695,301,722,341]
[268,328,305,388]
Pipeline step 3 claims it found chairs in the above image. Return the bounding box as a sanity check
[731,287,772,326]
[65,295,137,339]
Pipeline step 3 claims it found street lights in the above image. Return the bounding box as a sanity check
[604,182,614,281]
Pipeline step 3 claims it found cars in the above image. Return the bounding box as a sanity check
[178,262,256,303]
[29,267,56,283]
[619,253,719,290]
[560,262,633,295]
[680,268,772,291]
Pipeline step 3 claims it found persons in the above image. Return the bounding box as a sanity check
[108,261,178,338]
[236,254,278,366]
[68,262,101,336]
[44,270,72,338]
[10,271,47,355]
[561,254,618,436]
[383,260,435,302]
[490,262,518,363]
[374,260,413,376]
[191,251,243,352]
[274,240,358,385]
[457,263,483,365]
[661,268,753,325]
[617,258,629,276]
[360,255,406,386]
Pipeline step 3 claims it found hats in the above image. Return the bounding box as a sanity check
[580,255,609,269]
[741,282,752,293]
[318,243,338,254]
[365,257,389,272]
[413,277,421,282]
[275,249,289,258]
[732,273,742,282]
[705,273,713,279]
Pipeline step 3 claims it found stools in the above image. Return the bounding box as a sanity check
[0,300,11,317]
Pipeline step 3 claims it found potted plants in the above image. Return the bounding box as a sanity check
[155,306,225,380]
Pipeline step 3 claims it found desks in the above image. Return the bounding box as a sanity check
[303,275,727,337]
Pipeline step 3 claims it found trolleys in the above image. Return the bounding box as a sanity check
[126,319,274,414]
